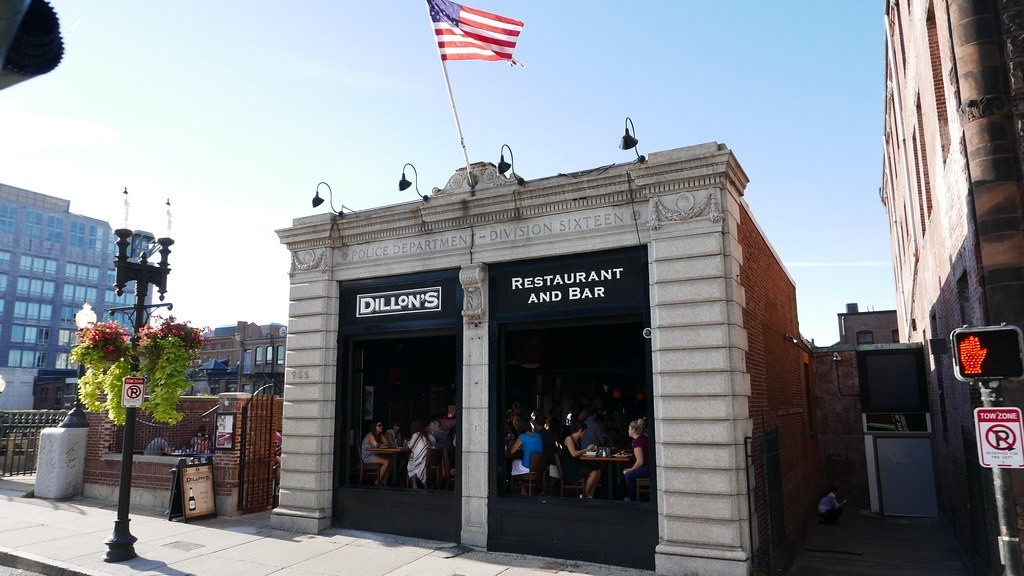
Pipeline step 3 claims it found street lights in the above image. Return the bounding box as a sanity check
[102,227,176,563]
[54,302,99,428]
[198,358,230,396]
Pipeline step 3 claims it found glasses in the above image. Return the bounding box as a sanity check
[376,424,383,428]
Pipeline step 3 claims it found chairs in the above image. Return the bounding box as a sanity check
[356,445,380,486]
[553,453,585,498]
[443,448,455,489]
[636,478,650,502]
[406,448,443,491]
[510,452,549,497]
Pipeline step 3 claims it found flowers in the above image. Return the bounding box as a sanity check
[68,319,133,427]
[133,313,213,428]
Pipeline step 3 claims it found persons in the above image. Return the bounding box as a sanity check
[817,484,849,526]
[361,421,393,488]
[189,424,212,454]
[384,422,402,482]
[428,420,452,449]
[143,431,172,455]
[504,402,650,502]
[404,419,430,488]
[272,432,282,480]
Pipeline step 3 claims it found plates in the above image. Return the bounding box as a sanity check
[582,453,599,457]
[612,454,632,457]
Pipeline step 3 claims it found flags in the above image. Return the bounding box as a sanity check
[427,0,524,67]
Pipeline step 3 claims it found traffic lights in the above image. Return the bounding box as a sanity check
[950,324,1024,382]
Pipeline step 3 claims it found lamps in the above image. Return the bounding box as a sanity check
[399,162,429,203]
[618,117,646,164]
[497,144,525,186]
[311,181,345,218]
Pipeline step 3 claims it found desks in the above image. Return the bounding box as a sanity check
[168,453,201,456]
[366,447,411,487]
[579,456,633,501]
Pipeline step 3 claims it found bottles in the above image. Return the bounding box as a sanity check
[598,450,602,458]
[602,450,606,458]
